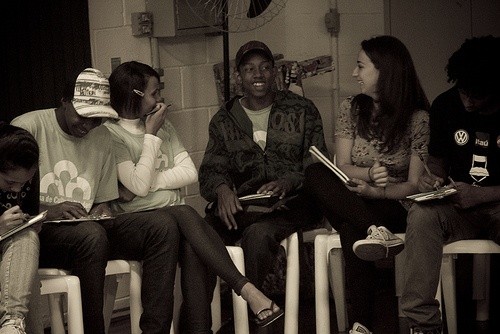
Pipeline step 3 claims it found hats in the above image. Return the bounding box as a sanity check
[236,41,273,67]
[64,66,119,119]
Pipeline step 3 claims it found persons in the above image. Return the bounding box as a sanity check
[400,35,500,334]
[197,41,329,334]
[304,35,431,334]
[103,61,284,334]
[0,125,40,334]
[11,68,180,334]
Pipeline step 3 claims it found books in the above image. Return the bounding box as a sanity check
[0,209,47,241]
[406,187,457,202]
[309,146,353,186]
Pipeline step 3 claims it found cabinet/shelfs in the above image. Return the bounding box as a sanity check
[144,0,224,37]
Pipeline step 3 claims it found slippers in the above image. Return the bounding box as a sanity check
[255,300,283,327]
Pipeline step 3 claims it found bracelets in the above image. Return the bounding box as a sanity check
[368,167,374,182]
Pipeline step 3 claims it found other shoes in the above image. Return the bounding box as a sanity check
[1,318,26,334]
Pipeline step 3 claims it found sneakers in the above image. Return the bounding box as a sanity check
[351,225,406,260]
[349,321,372,334]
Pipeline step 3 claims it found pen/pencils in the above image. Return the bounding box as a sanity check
[145,103,173,116]
[417,152,437,189]
[447,176,457,187]
[0,202,28,221]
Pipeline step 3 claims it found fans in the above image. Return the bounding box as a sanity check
[186,0,288,103]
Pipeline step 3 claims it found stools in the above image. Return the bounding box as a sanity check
[28,227,500,334]
